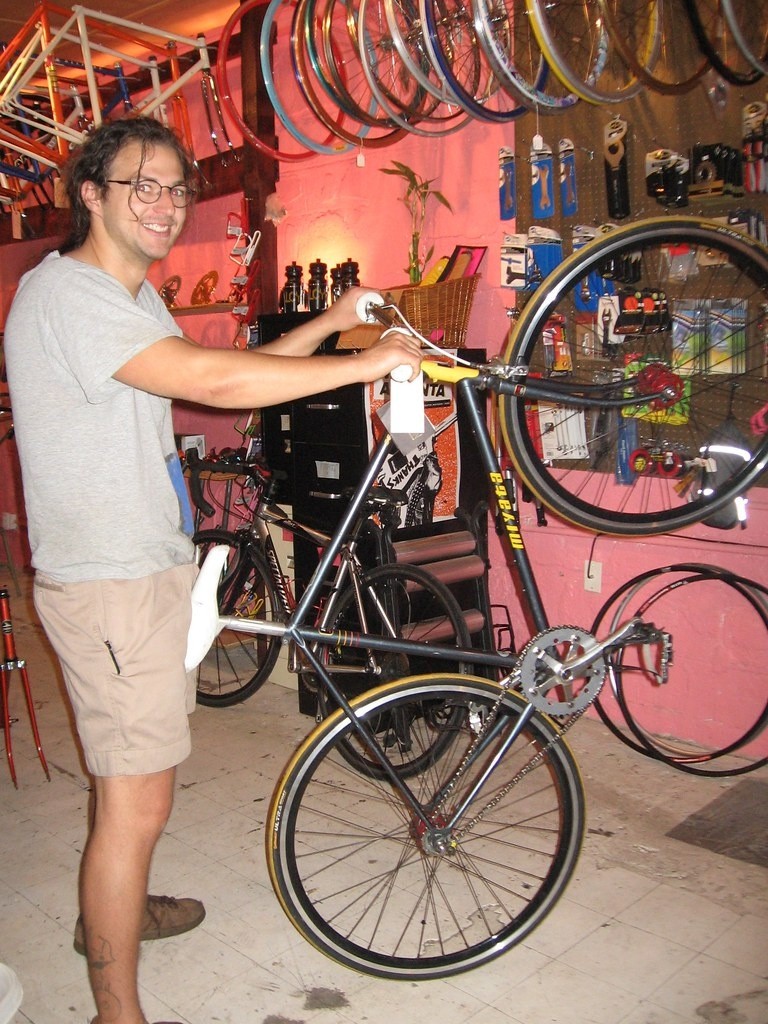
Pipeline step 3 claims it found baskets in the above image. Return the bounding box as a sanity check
[381,273,480,348]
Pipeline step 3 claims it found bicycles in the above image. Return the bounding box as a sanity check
[184,215,768,981]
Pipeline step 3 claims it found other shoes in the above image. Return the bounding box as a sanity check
[73,894,206,956]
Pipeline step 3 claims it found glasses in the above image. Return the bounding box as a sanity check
[101,178,197,208]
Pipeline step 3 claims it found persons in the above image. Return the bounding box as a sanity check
[4,114,426,1024]
